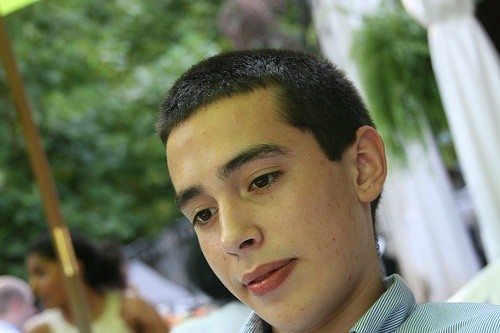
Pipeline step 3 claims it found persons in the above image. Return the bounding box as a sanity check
[159,47,500,333]
[23,233,168,333]
[102,244,194,312]
[1,275,39,333]
